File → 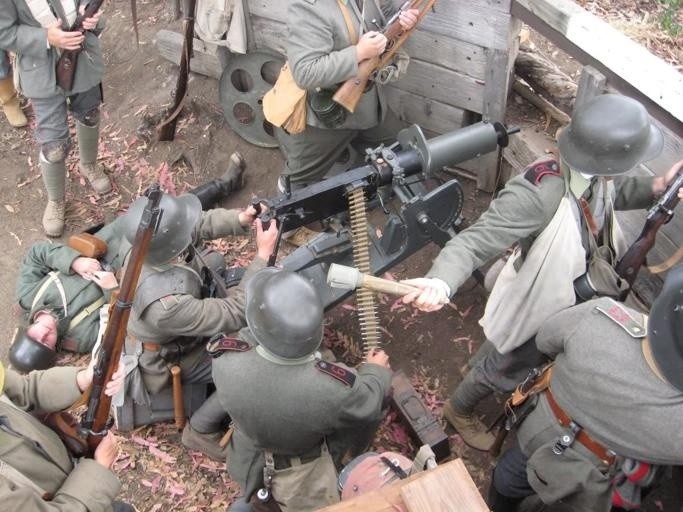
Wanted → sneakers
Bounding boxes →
[78,160,112,195]
[487,467,526,512]
[181,419,228,463]
[280,225,321,247]
[442,398,497,452]
[42,198,65,236]
[460,363,471,380]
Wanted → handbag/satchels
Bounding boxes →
[477,189,588,355]
[263,437,341,512]
[91,304,153,409]
[526,419,614,512]
[261,60,308,135]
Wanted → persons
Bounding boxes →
[402,95,683,511]
[0,192,395,511]
[1,0,112,236]
[273,1,421,246]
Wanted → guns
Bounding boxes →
[158,1,197,140]
[75,184,165,456]
[612,165,683,304]
[331,0,437,114]
[54,0,104,93]
[249,115,521,313]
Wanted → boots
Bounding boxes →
[187,150,246,211]
[0,74,28,127]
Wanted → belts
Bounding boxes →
[126,334,162,351]
[543,385,620,468]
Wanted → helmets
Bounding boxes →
[244,265,326,363]
[646,261,683,393]
[556,93,665,177]
[122,192,202,267]
[8,325,55,372]
